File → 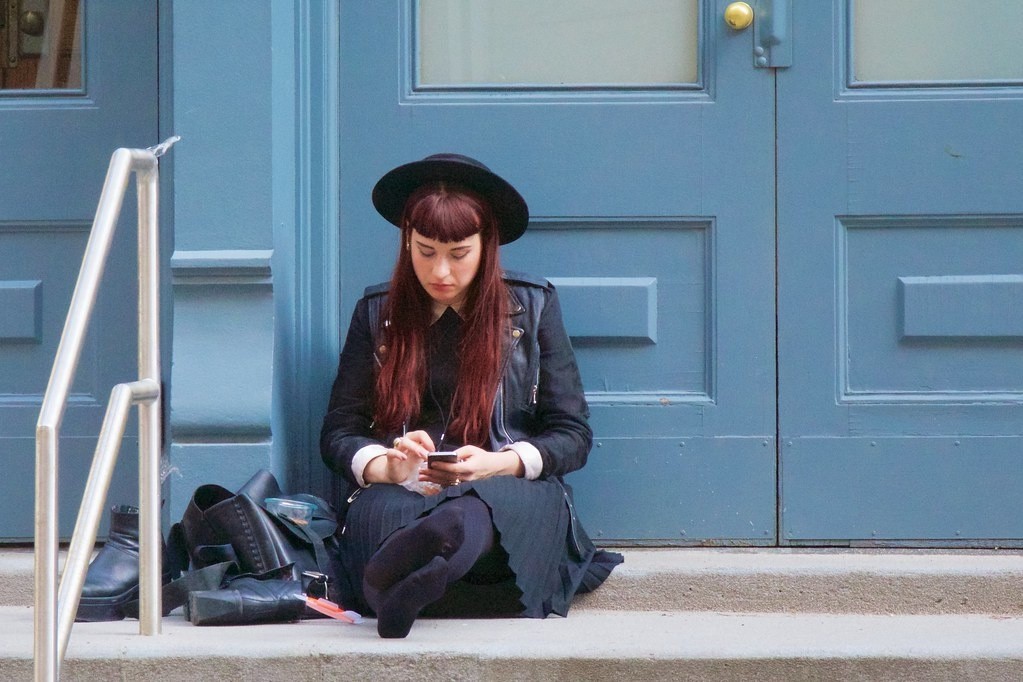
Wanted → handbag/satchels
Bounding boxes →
[168,472,356,613]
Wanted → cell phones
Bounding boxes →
[428,450,458,476]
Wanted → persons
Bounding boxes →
[320,154,624,639]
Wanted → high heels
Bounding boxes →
[182,562,307,625]
[74,505,172,622]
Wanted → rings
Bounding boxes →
[393,438,400,447]
[450,472,461,486]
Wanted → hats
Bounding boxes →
[371,153,529,246]
